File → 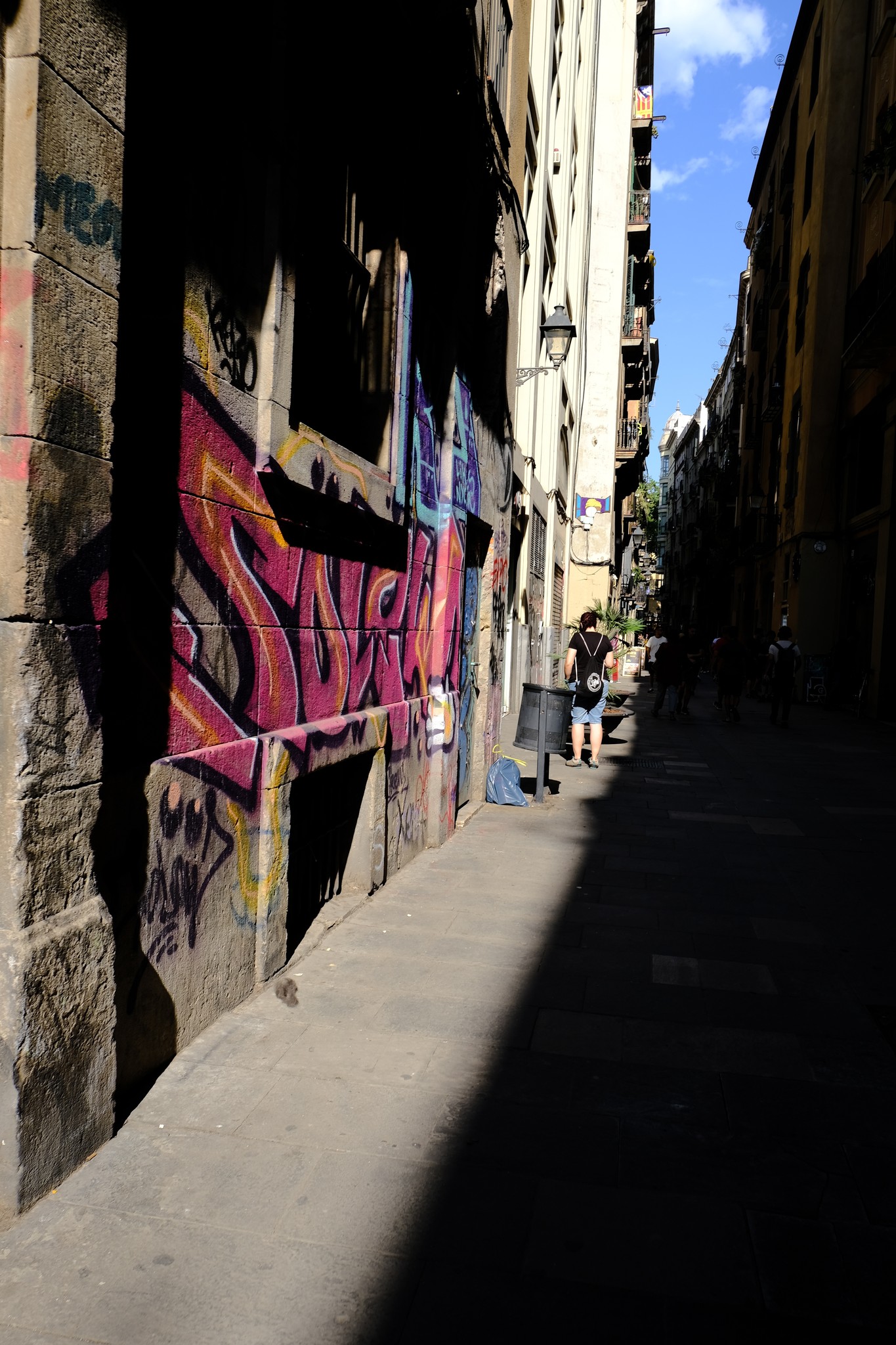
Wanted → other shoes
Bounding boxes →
[675,708,681,714]
[713,701,723,710]
[647,687,654,693]
[651,708,658,717]
[681,707,691,715]
[668,713,677,721]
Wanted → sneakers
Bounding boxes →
[566,756,582,768]
[588,757,599,769]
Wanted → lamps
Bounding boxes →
[636,551,651,566]
[513,303,577,385]
[625,524,645,553]
[641,570,653,581]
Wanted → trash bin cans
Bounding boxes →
[513,682,577,754]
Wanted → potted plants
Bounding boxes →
[544,596,647,743]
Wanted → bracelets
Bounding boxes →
[564,679,569,682]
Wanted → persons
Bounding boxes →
[638,624,802,727]
[564,611,613,769]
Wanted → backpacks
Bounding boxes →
[772,641,797,680]
[575,632,605,700]
